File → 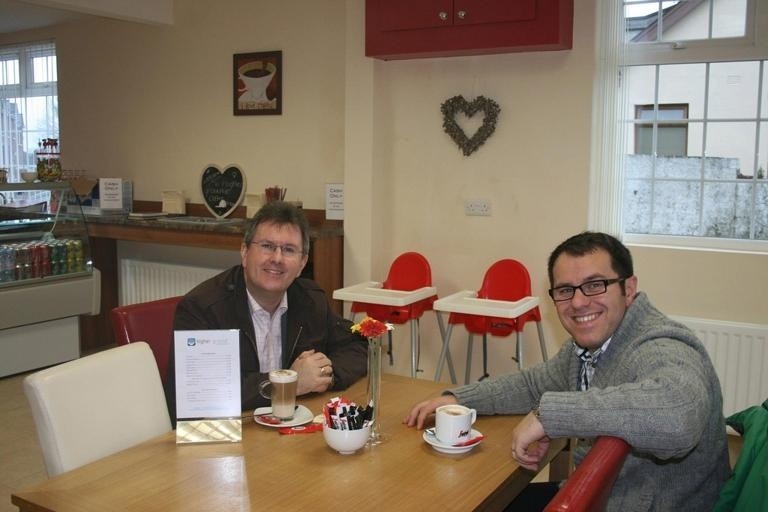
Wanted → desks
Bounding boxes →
[70,206,343,321]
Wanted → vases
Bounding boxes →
[367,336,383,439]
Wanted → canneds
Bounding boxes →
[0,239,93,282]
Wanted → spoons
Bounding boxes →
[232,404,298,421]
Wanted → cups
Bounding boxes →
[434,404,477,441]
[258,368,298,419]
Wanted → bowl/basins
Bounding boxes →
[321,419,375,454]
[21,172,38,183]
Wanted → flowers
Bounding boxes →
[348,316,389,430]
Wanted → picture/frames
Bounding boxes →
[231,50,283,116]
[161,188,186,216]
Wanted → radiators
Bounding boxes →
[659,312,768,435]
[117,257,226,309]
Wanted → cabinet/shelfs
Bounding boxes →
[363,0,575,64]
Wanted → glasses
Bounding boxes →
[547,276,625,303]
[244,238,305,258]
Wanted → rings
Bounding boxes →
[320,367,326,375]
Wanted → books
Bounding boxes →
[128,212,168,217]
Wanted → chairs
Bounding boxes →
[432,258,547,385]
[332,250,458,385]
[498,430,629,511]
[21,340,174,478]
[108,293,190,384]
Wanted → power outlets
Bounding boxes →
[465,200,490,216]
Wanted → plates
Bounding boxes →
[253,403,313,429]
[422,427,482,453]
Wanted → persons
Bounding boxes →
[401,232,735,512]
[164,202,371,428]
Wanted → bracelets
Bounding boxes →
[531,403,543,422]
